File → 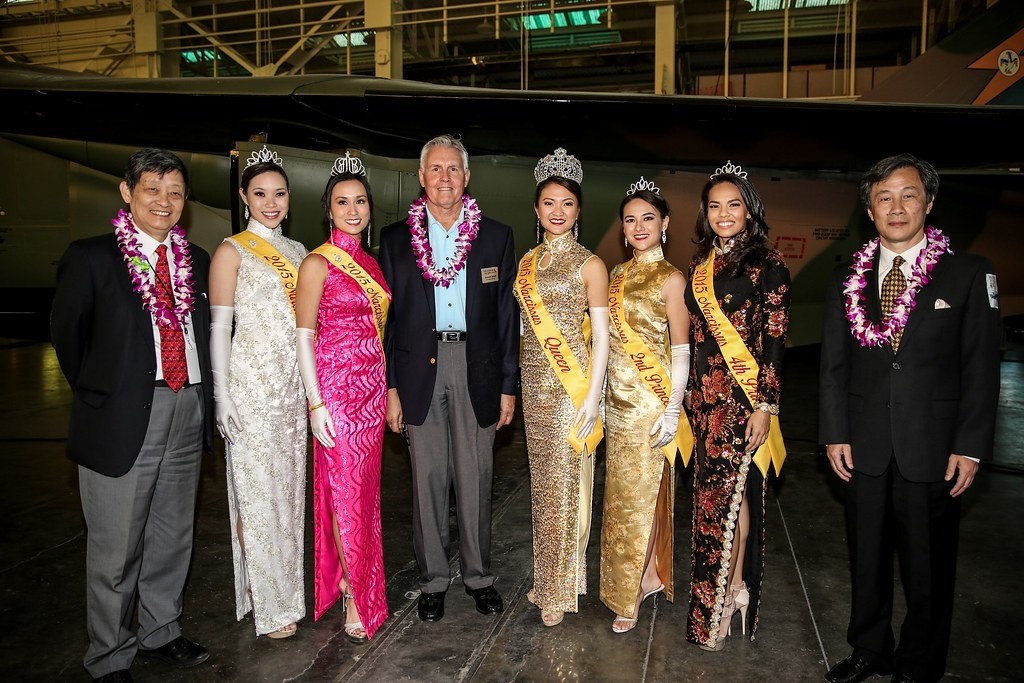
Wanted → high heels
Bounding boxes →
[247,590,255,619]
[339,578,351,612]
[730,581,749,635]
[612,591,644,633]
[699,587,736,651]
[344,594,366,642]
[643,584,665,608]
[266,622,297,638]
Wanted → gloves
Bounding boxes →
[574,307,609,439]
[295,328,336,448]
[209,306,242,444]
[650,344,690,449]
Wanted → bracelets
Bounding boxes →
[309,403,324,411]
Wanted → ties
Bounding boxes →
[881,255,909,356]
[156,244,188,392]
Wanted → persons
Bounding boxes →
[513,147,608,625]
[819,155,999,683]
[210,145,309,638]
[52,149,210,683]
[379,137,519,622]
[600,177,692,634]
[297,153,391,642]
[683,161,791,650]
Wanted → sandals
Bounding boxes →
[541,609,564,627]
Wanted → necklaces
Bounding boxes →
[112,210,195,327]
[843,225,950,345]
[409,194,481,287]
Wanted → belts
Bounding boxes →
[437,331,466,342]
[154,379,201,389]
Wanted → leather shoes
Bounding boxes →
[465,586,503,616]
[891,673,932,683]
[418,592,446,622]
[138,637,210,666]
[94,669,133,683]
[825,655,893,683]
